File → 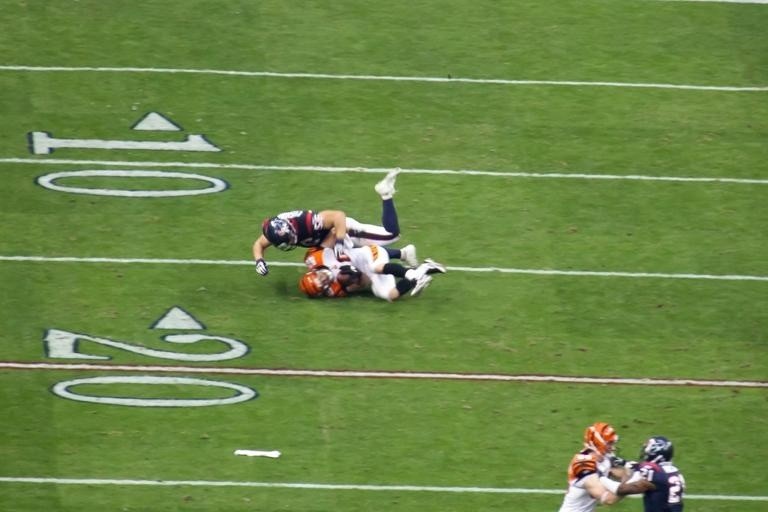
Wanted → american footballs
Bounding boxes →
[336,270,360,285]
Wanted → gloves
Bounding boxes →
[255,259,269,276]
[333,240,343,260]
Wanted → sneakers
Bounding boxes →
[402,244,417,269]
[410,274,431,297]
[421,259,445,273]
[374,167,401,196]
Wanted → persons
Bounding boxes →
[558,422,644,512]
[599,434,686,512]
[252,167,447,304]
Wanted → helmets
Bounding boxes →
[261,216,297,251]
[638,436,672,464]
[298,265,334,299]
[582,422,618,460]
